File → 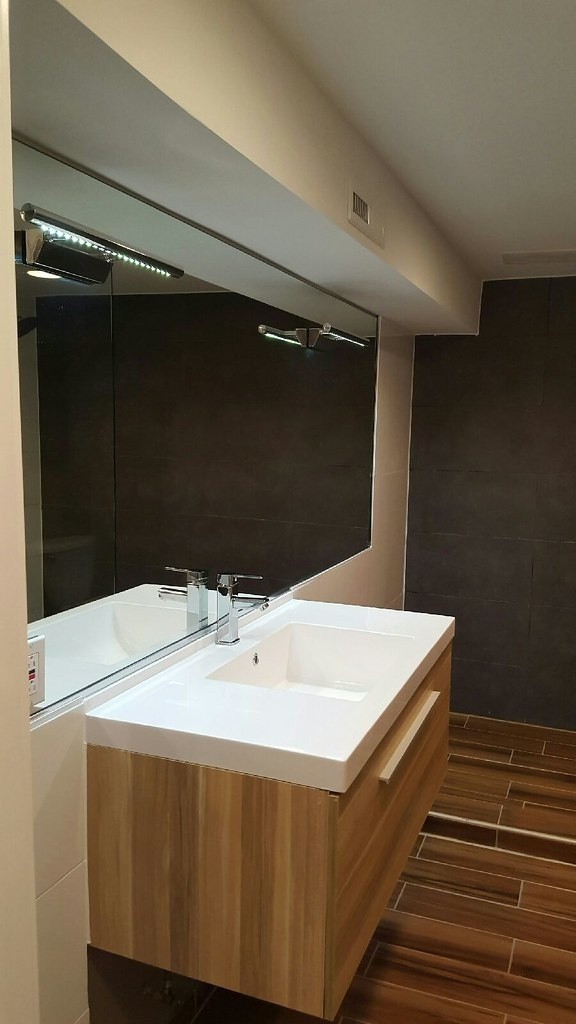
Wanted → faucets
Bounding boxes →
[215,574,269,646]
[158,567,208,636]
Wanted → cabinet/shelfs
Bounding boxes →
[88,642,452,1021]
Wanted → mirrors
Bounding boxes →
[12,137,380,717]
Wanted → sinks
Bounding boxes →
[204,621,417,703]
[27,601,215,667]
